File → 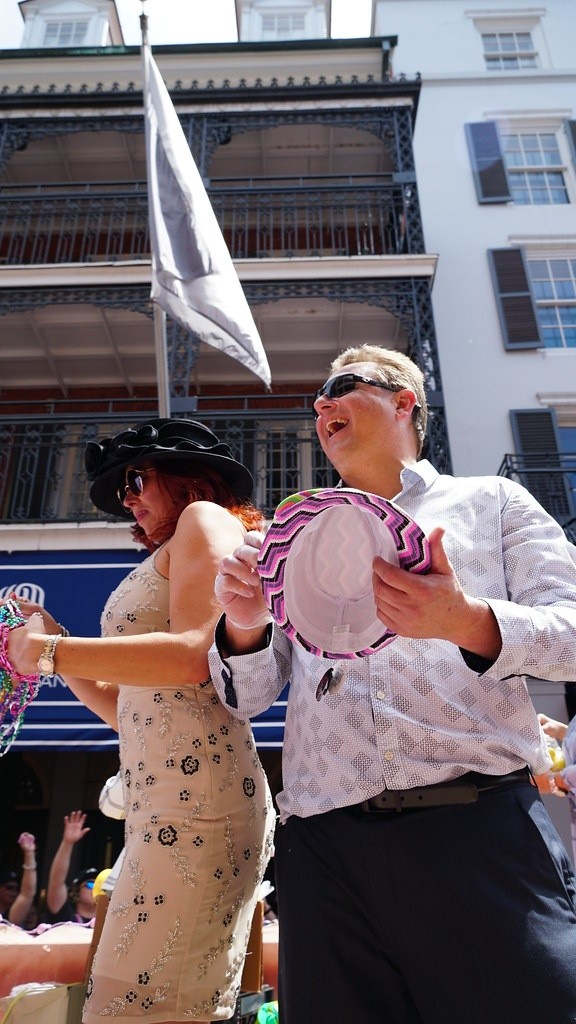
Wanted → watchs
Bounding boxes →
[37,634,61,678]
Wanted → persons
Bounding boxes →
[207,345,576,1023]
[8,415,276,1024]
[48,810,104,931]
[17,904,39,932]
[0,833,38,928]
[533,710,576,875]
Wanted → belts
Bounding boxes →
[343,767,532,813]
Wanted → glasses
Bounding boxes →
[2,884,20,891]
[116,466,158,514]
[310,373,423,420]
[78,880,94,888]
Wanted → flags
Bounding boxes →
[145,42,273,391]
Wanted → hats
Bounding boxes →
[0,870,20,886]
[71,865,100,887]
[253,488,432,662]
[83,416,254,521]
